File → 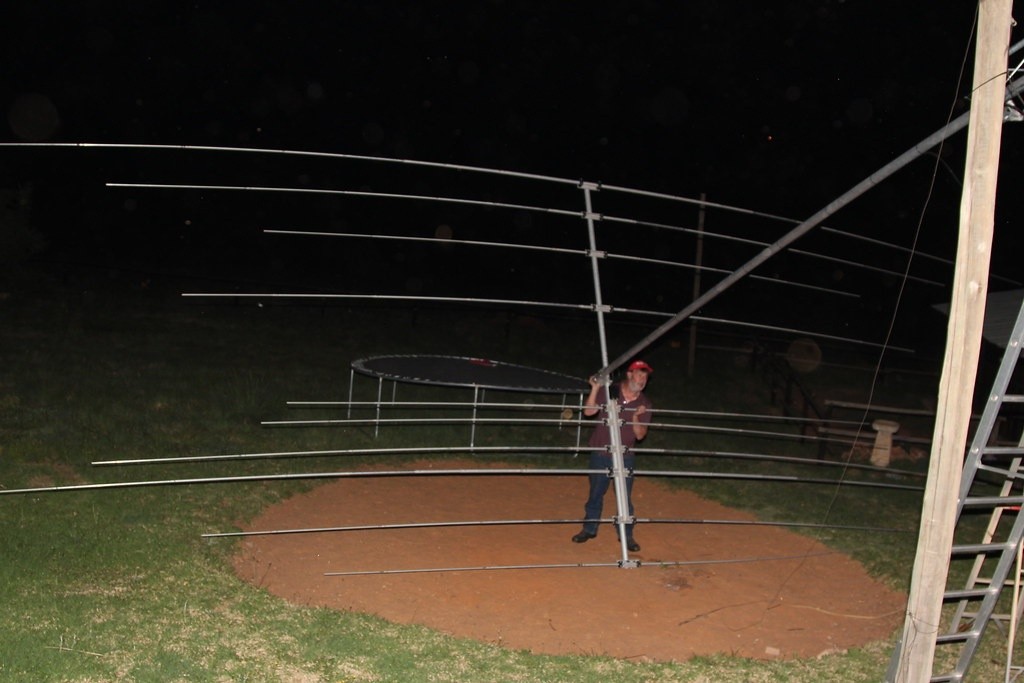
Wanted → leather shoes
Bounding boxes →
[618,538,640,551]
[572,531,596,543]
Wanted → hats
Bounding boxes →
[628,361,652,373]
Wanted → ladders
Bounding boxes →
[884,300,1024,683]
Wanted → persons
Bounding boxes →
[583,361,653,554]
